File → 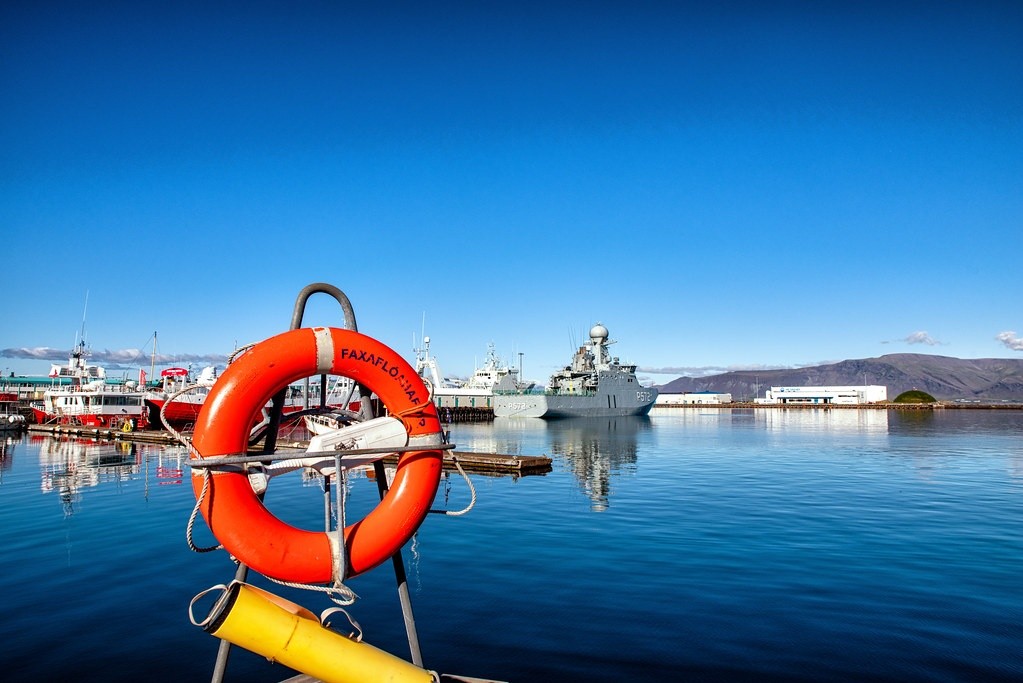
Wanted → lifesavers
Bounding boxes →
[189,326,444,585]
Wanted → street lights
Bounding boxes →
[517,352,526,383]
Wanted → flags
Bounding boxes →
[140,369,147,385]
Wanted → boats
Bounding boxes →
[0,285,535,438]
[491,320,659,419]
